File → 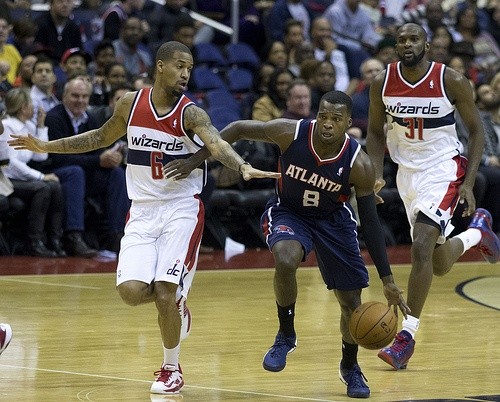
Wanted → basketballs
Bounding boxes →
[349,301,398,350]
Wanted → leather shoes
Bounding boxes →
[46,238,67,257]
[15,240,57,258]
[62,232,97,258]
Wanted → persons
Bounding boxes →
[5,41,282,395]
[365,23,499,368]
[0,0,496,255]
[161,90,410,398]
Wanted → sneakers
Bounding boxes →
[378,330,415,369]
[469,208,500,264]
[176,296,191,338]
[150,362,184,394]
[338,359,370,398]
[262,328,298,372]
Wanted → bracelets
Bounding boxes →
[236,161,252,174]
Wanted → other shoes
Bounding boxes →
[0,323,12,356]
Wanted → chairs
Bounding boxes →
[0,0,488,261]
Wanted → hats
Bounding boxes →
[62,47,93,65]
